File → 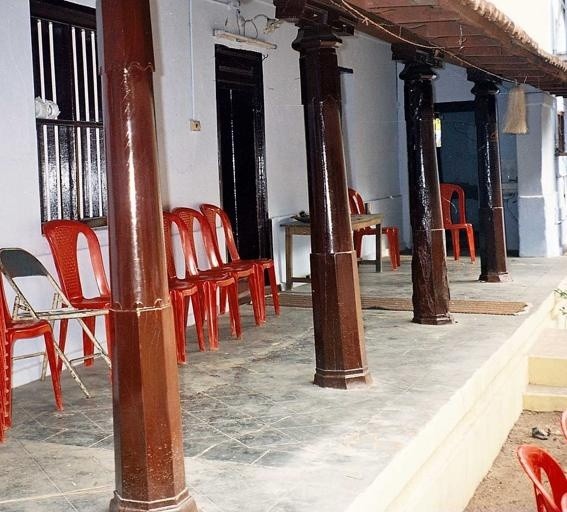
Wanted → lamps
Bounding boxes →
[244,14,285,35]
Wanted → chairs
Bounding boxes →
[0,247,112,399]
[42,219,113,375]
[0,275,64,442]
[348,187,400,271]
[517,445,567,512]
[560,411,567,440]
[440,183,475,262]
[162,204,279,364]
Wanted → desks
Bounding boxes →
[279,213,383,290]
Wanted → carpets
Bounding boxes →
[251,292,529,316]
